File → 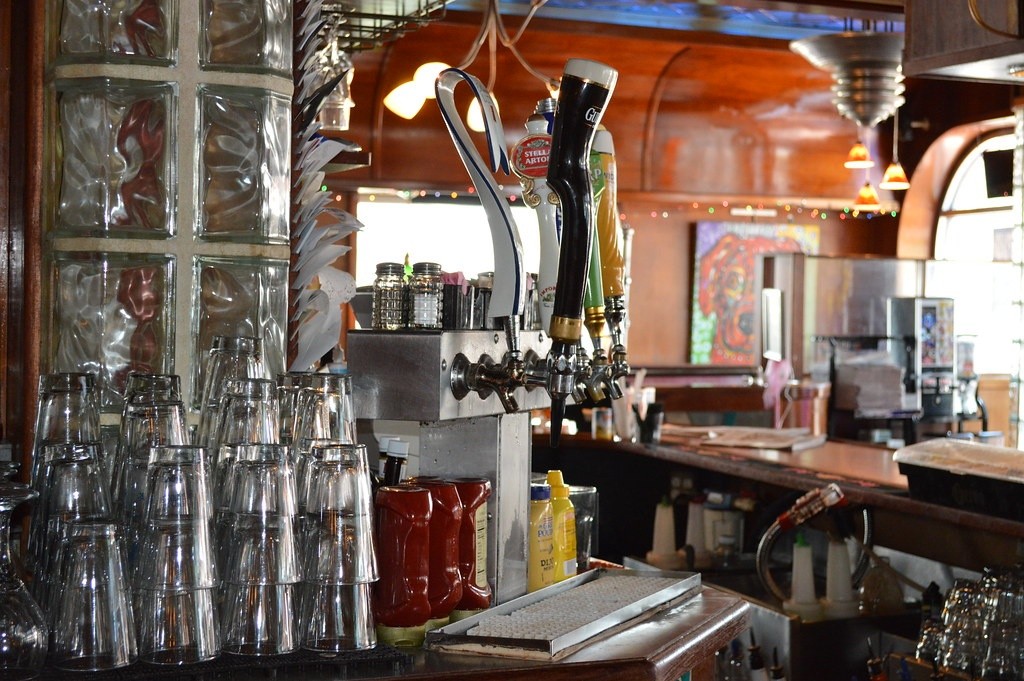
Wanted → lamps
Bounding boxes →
[382,0,560,133]
[788,15,912,211]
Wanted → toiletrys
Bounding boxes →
[527,469,578,592]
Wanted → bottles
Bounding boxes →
[826,531,852,602]
[686,492,705,552]
[408,262,444,329]
[728,631,787,681]
[370,435,414,500]
[791,531,815,604]
[654,496,676,555]
[372,263,406,329]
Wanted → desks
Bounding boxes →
[530,410,1024,565]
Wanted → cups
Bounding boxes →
[0,364,378,675]
[856,557,903,612]
[565,486,600,574]
[915,566,1024,681]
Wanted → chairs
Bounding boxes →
[974,378,1012,431]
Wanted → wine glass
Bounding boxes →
[320,4,355,109]
[319,26,353,86]
[321,14,350,131]
